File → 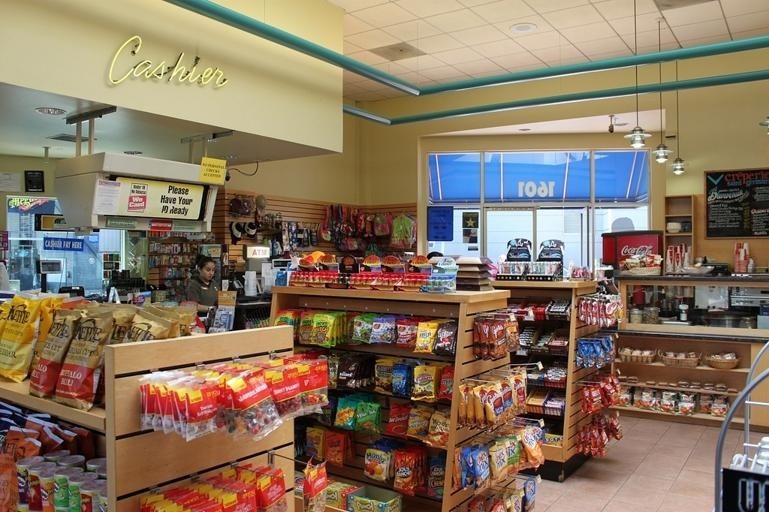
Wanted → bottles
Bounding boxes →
[182,243,191,253]
[496,261,561,276]
[290,269,455,289]
[12,448,108,512]
[166,266,173,278]
[626,284,694,324]
[738,316,756,329]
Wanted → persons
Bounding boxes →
[426,251,443,260]
[184,252,222,316]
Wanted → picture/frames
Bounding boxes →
[24,169,45,193]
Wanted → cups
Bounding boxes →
[732,242,755,274]
[109,269,145,291]
[680,221,692,230]
[665,243,691,273]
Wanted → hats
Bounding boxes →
[229,221,258,241]
[254,194,267,217]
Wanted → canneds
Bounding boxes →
[679,304,689,321]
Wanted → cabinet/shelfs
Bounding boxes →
[714,342,769,511]
[0,251,627,512]
[661,192,697,274]
[596,232,769,433]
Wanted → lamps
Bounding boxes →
[670,50,686,176]
[653,19,673,165]
[621,0,653,149]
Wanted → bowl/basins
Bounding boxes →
[754,266,768,273]
[666,222,681,233]
